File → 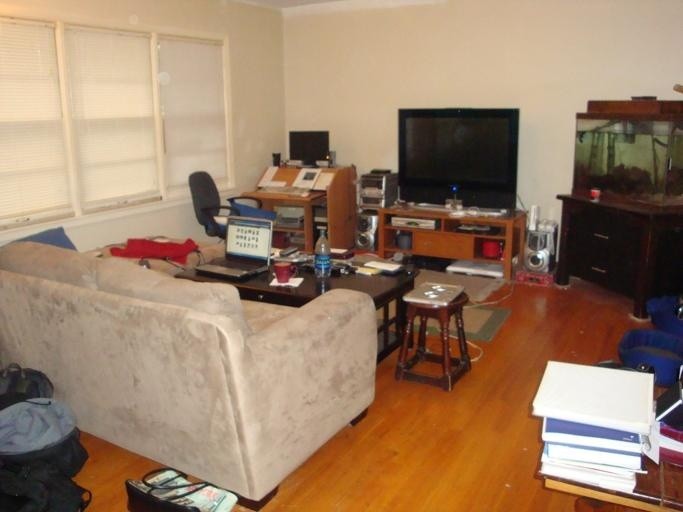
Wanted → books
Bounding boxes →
[533,359,682,492]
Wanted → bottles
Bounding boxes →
[312,229,331,278]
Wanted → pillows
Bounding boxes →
[7,226,78,252]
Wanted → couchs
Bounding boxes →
[0,240,379,510]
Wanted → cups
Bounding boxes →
[589,188,602,204]
[273,263,291,282]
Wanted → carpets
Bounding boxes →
[412,268,513,348]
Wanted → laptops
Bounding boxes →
[194,215,275,281]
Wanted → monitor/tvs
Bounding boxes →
[289,130,329,168]
[397,107,520,209]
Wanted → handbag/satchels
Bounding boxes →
[125,467,239,512]
[0,364,92,512]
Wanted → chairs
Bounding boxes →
[186,169,278,238]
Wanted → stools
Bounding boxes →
[391,282,474,395]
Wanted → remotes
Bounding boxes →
[279,246,299,256]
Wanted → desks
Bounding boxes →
[533,424,683,511]
[552,192,683,323]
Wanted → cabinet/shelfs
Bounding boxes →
[248,162,525,277]
